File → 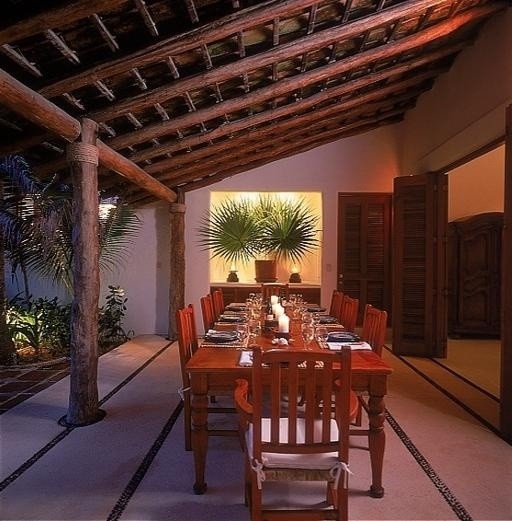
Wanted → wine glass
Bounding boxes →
[249,321,260,347]
[236,324,248,350]
[316,326,328,349]
[289,293,321,324]
[239,293,262,323]
[301,323,315,351]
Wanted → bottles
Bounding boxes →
[278,295,287,307]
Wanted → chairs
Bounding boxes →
[298,290,387,427]
[175,289,248,452]
[233,345,352,521]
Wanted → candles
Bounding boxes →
[270,295,289,332]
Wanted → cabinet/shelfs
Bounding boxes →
[210,284,321,311]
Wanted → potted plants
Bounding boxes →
[193,192,323,282]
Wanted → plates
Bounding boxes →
[225,305,246,311]
[308,307,326,313]
[328,331,360,342]
[203,330,239,342]
[220,314,244,323]
[315,314,338,324]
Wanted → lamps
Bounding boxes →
[288,261,302,283]
[226,261,240,282]
